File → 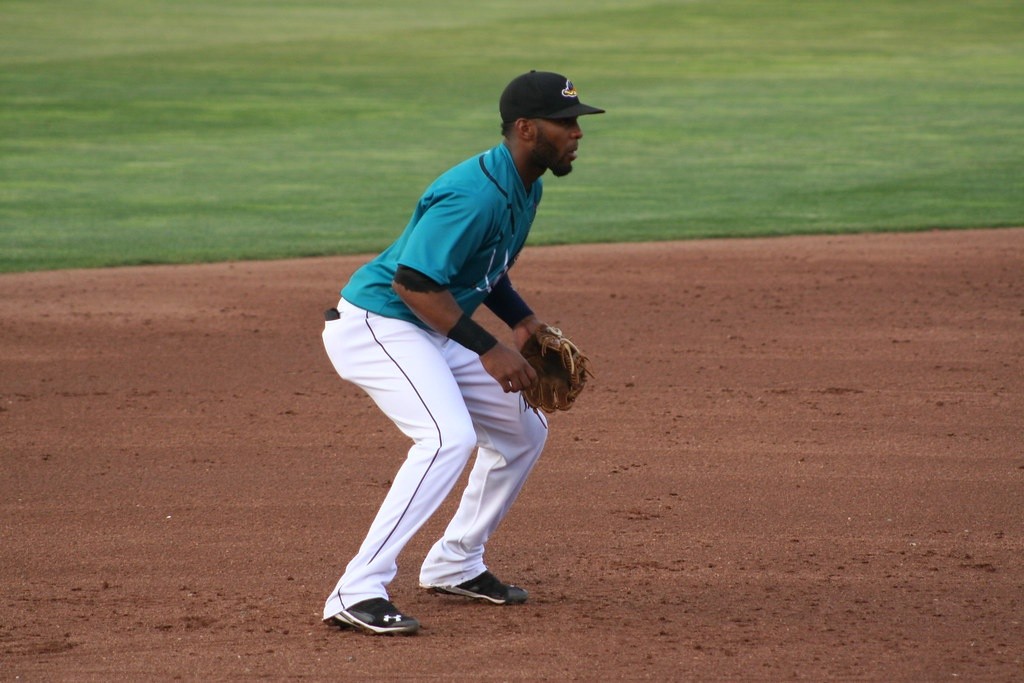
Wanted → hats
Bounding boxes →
[500,70,605,123]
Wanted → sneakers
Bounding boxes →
[434,569,528,604]
[322,598,419,636]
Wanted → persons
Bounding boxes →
[322,69,606,636]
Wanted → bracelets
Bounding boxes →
[447,312,499,356]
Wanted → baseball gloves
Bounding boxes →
[516,322,588,415]
